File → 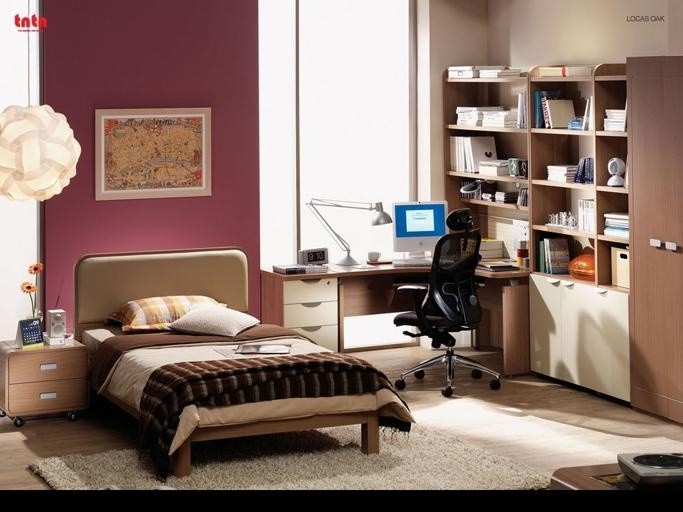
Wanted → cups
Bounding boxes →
[368,251,381,262]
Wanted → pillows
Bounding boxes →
[106,295,260,337]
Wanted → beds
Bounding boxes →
[75,246,416,477]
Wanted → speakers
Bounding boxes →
[48,308,66,338]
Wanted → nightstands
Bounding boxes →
[0,338,90,428]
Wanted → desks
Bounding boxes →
[551,463,683,490]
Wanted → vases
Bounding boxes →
[26,309,44,323]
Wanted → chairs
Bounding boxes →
[394,208,501,397]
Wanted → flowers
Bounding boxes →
[21,262,43,318]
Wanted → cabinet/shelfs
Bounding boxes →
[530,64,630,404]
[260,261,532,378]
[442,69,530,268]
[626,57,683,425]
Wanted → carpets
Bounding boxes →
[28,423,551,490]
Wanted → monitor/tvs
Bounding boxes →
[392,200,449,258]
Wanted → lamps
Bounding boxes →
[1,1,82,201]
[305,198,392,265]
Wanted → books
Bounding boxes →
[272,264,327,275]
[447,66,630,291]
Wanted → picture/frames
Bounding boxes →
[95,108,211,201]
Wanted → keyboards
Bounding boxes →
[392,258,432,267]
